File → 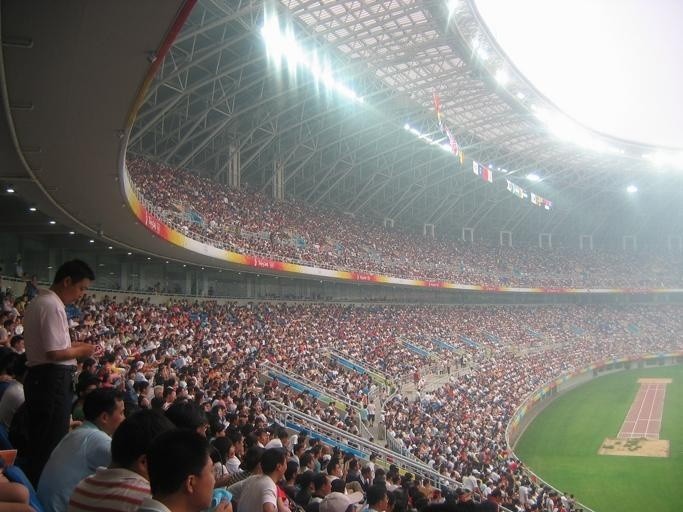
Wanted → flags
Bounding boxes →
[432,92,464,164]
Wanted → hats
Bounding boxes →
[317,491,366,512]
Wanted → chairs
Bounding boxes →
[5,464,45,512]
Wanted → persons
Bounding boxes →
[127,155,681,261]
[0,260,683,512]
